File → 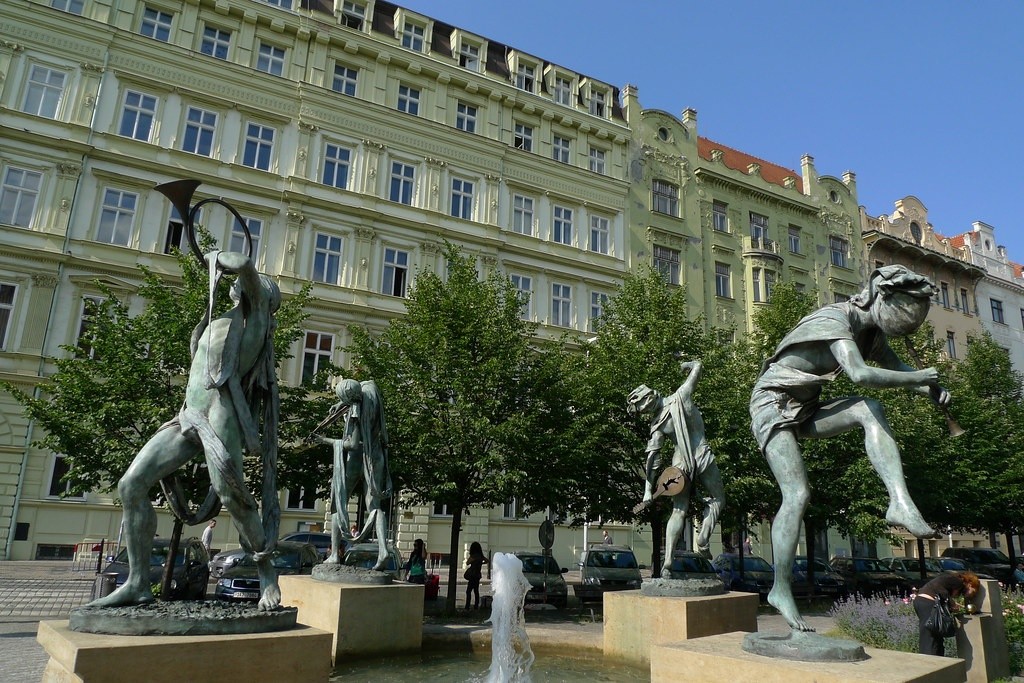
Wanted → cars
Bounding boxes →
[770,555,846,601]
[213,541,324,606]
[514,551,569,611]
[878,546,1024,592]
[208,532,371,577]
[709,552,774,596]
[651,550,722,583]
[828,556,909,601]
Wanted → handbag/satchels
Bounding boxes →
[925,594,957,637]
[463,568,482,580]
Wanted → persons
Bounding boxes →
[85,248,285,611]
[462,541,489,613]
[200,518,216,558]
[403,538,432,590]
[749,263,950,632]
[602,529,614,556]
[627,361,727,578]
[323,540,358,565]
[312,379,393,571]
[914,572,980,656]
[732,538,752,554]
[1014,562,1024,596]
[351,525,361,539]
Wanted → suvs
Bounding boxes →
[341,543,404,581]
[90,534,210,604]
[577,544,646,602]
